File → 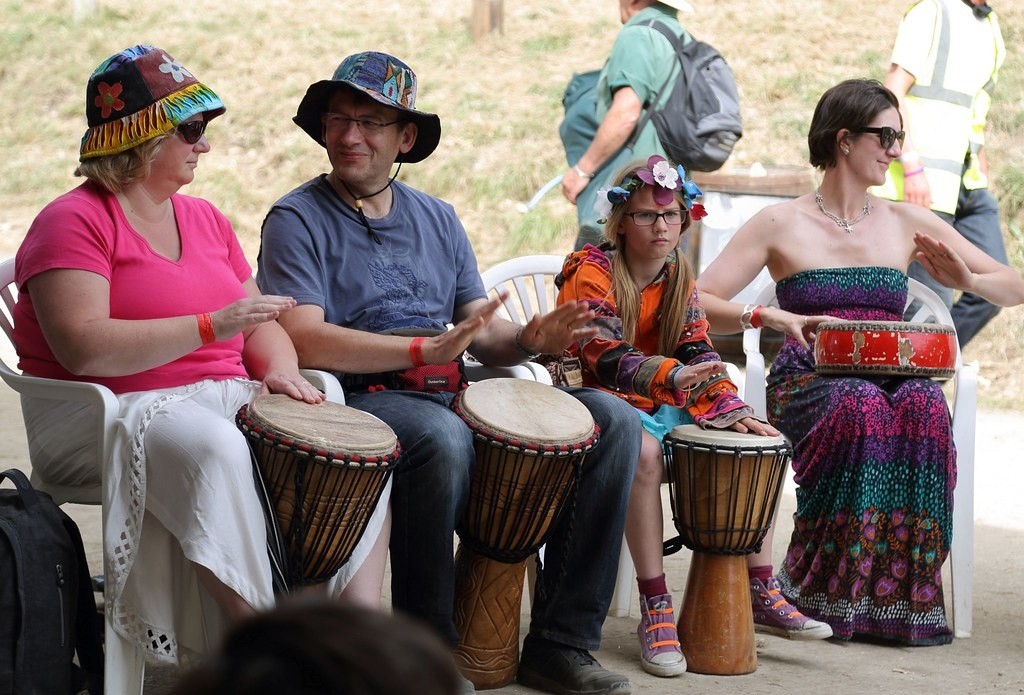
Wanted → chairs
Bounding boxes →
[0,257,346,695]
[468,256,741,618]
[743,276,979,640]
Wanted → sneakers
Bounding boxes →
[515,632,634,695]
[635,593,687,678]
[749,576,834,641]
[443,649,477,694]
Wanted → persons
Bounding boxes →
[870,0,1008,388]
[254,50,642,695]
[694,78,1024,647]
[562,0,695,252]
[12,42,392,668]
[554,154,833,676]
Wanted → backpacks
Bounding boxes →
[0,466,105,695]
[632,18,745,173]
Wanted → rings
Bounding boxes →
[943,254,947,258]
[568,325,572,331]
[805,317,807,325]
[303,382,309,384]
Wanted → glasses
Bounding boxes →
[623,209,689,227]
[851,126,905,151]
[320,111,402,134]
[168,120,212,145]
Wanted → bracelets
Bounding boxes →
[740,304,763,329]
[409,337,427,367]
[669,365,701,391]
[515,325,541,359]
[904,167,923,177]
[196,313,216,346]
[575,166,590,178]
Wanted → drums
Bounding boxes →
[663,423,787,675]
[237,392,401,608]
[452,378,602,686]
[814,319,956,379]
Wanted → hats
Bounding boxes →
[657,0,695,13]
[78,43,228,162]
[291,50,443,164]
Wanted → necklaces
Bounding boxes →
[815,190,871,234]
